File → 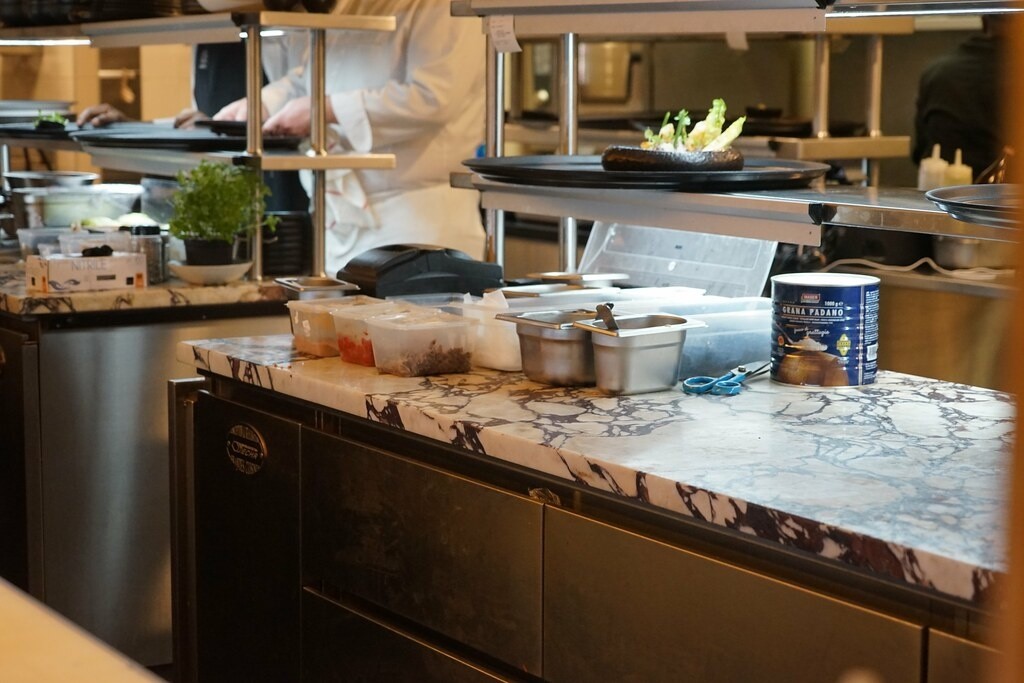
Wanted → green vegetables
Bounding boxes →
[645,100,746,152]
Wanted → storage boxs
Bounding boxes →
[274,276,775,398]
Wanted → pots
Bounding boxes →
[12,184,146,230]
[3,171,101,191]
[935,234,1018,269]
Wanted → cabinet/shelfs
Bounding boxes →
[0,317,1021,683]
[1,0,1024,289]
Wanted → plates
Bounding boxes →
[167,259,254,284]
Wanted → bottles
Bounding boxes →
[938,149,972,187]
[974,144,1015,185]
[917,144,948,191]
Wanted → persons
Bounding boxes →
[77,0,313,272]
[910,14,1006,190]
[211,0,500,281]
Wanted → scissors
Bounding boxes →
[682,361,770,396]
